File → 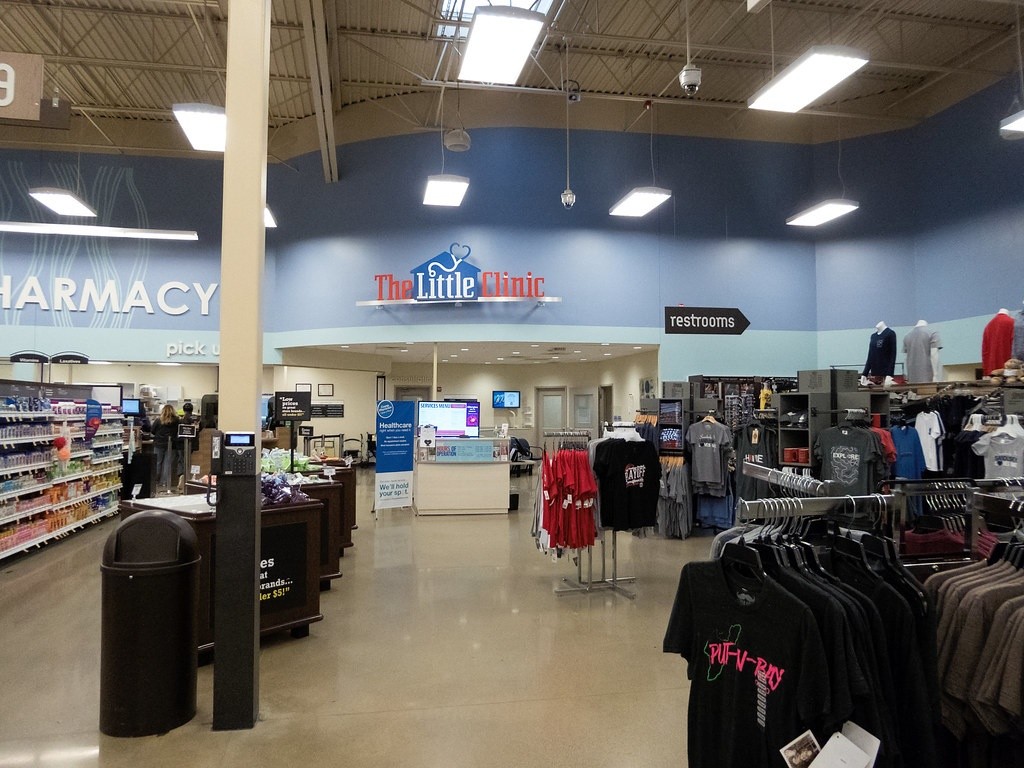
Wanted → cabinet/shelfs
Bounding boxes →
[0,410,125,561]
[690,381,724,423]
[777,369,834,467]
[833,369,890,434]
[657,381,691,450]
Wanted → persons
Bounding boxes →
[901,319,943,382]
[861,321,897,387]
[982,301,1024,381]
[151,403,198,490]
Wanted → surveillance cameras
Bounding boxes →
[561,190,576,210]
[678,63,701,95]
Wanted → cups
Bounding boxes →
[502,423,508,437]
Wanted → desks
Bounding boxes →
[118,460,363,668]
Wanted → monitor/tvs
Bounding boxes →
[492,391,520,408]
[418,401,481,439]
[122,399,140,414]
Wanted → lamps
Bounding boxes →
[443,79,472,152]
[173,0,226,152]
[264,204,278,228]
[0,221,199,240]
[423,95,469,207]
[998,94,1024,141]
[746,4,870,113]
[28,110,98,216]
[455,6,545,86]
[784,119,859,227]
[609,95,672,216]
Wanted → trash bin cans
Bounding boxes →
[99,509,202,738]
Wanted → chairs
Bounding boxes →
[342,432,376,468]
[511,437,543,478]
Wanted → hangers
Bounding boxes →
[543,431,585,458]
[603,421,645,442]
[702,410,717,423]
[961,396,1024,438]
[633,409,657,427]
[838,409,873,431]
[923,385,955,414]
[659,451,686,467]
[721,467,1024,583]
[897,418,909,429]
[730,408,777,436]
